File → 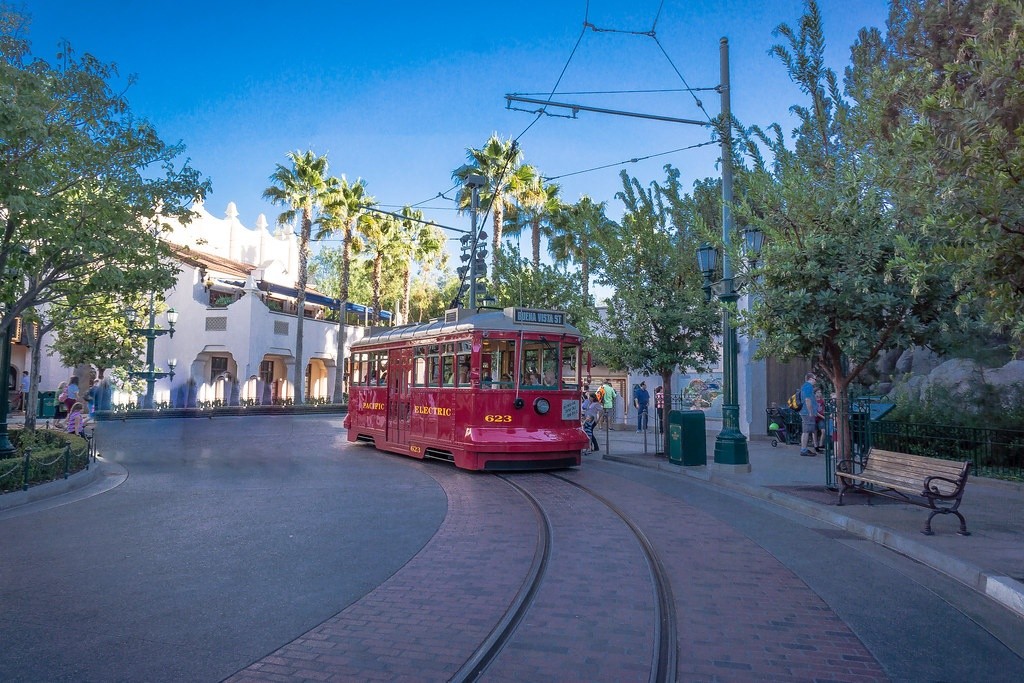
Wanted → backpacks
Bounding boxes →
[58,384,70,402]
[787,389,803,411]
[594,386,606,403]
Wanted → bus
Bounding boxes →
[339,141,592,473]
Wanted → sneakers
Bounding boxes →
[800,449,817,456]
[813,445,826,454]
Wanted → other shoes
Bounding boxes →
[599,427,604,431]
[642,429,652,434]
[584,450,592,455]
[636,429,641,434]
[608,427,615,432]
[594,449,600,453]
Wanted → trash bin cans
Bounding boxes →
[667,410,707,466]
[36,391,58,419]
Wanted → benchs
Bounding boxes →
[834,445,973,536]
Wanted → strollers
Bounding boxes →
[765,407,798,447]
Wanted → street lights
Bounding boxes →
[126,217,178,408]
[457,174,488,308]
[678,36,765,464]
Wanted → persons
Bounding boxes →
[52,381,68,429]
[635,381,651,434]
[500,373,513,389]
[371,369,387,383]
[448,361,470,383]
[582,392,604,456]
[17,371,30,410]
[88,379,100,416]
[64,375,82,413]
[656,386,664,434]
[597,380,616,431]
[798,373,825,457]
[67,402,90,439]
[545,369,557,386]
[525,372,541,385]
